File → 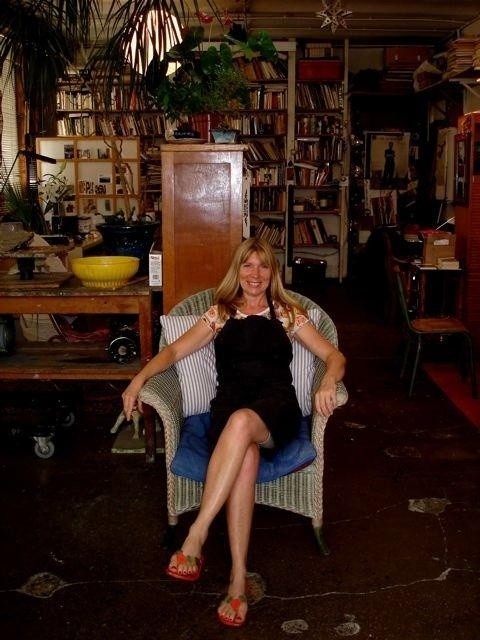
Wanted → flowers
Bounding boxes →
[34,158,77,216]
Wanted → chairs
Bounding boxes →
[136,281,351,561]
[392,270,475,399]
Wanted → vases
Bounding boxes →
[50,213,81,241]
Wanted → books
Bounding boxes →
[50,90,197,215]
[434,257,460,270]
[217,43,344,247]
[442,36,480,81]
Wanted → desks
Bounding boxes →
[391,252,466,348]
[0,272,160,465]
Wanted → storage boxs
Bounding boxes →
[147,241,162,289]
[421,233,458,268]
[298,58,343,83]
[384,43,433,72]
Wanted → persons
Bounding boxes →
[114,236,348,630]
[381,141,396,181]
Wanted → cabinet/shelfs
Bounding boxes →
[23,27,351,287]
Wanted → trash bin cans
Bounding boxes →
[292,256,327,303]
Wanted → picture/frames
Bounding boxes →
[453,133,470,204]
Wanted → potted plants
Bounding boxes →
[0,2,235,278]
[199,65,253,145]
[139,21,283,143]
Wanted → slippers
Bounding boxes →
[166,551,205,581]
[216,594,248,627]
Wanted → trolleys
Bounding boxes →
[0,380,77,460]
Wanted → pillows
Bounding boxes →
[159,307,325,421]
